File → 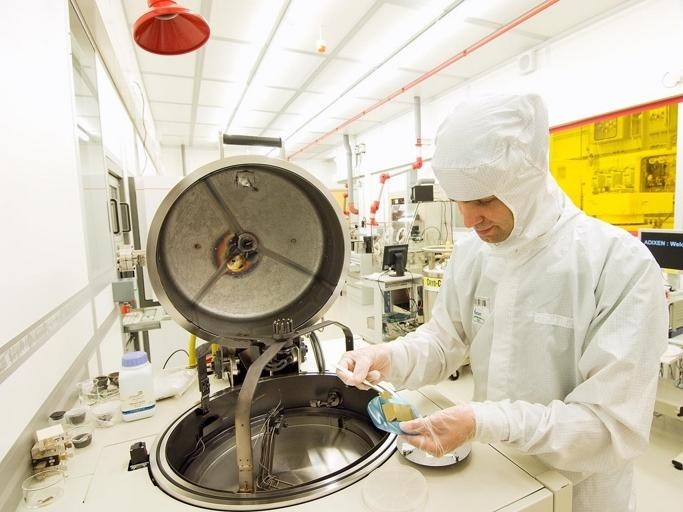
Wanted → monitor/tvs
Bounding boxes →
[637,229,682,274]
[382,244,408,277]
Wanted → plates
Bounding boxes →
[397,437,472,468]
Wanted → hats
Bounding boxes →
[426,92,583,271]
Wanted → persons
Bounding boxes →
[336,92,670,510]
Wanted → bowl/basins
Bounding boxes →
[20,467,68,512]
[67,371,119,450]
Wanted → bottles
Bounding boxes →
[118,350,157,424]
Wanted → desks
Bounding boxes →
[357,270,423,344]
